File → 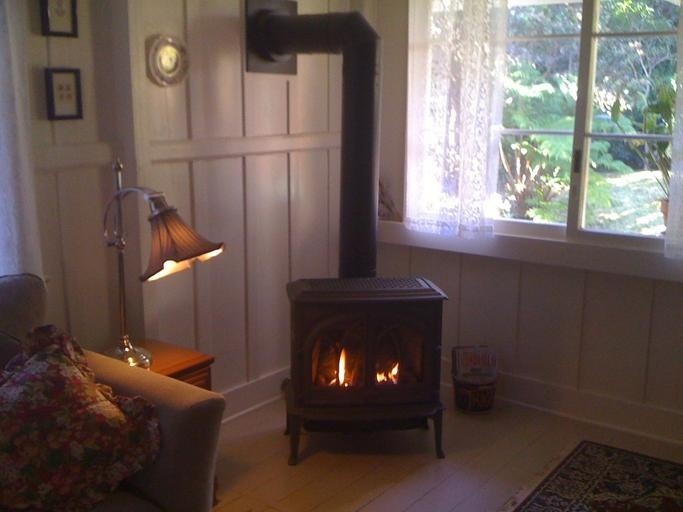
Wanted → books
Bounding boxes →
[453,345,496,374]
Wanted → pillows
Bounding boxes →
[1,321,162,510]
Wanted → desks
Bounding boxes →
[78,337,218,394]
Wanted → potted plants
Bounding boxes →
[609,74,671,234]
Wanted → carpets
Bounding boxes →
[510,436,682,512]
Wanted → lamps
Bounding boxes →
[96,157,226,376]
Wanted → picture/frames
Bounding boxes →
[40,0,78,37]
[45,68,83,120]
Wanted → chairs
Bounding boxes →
[0,269,229,512]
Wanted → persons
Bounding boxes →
[645,179,668,237]
